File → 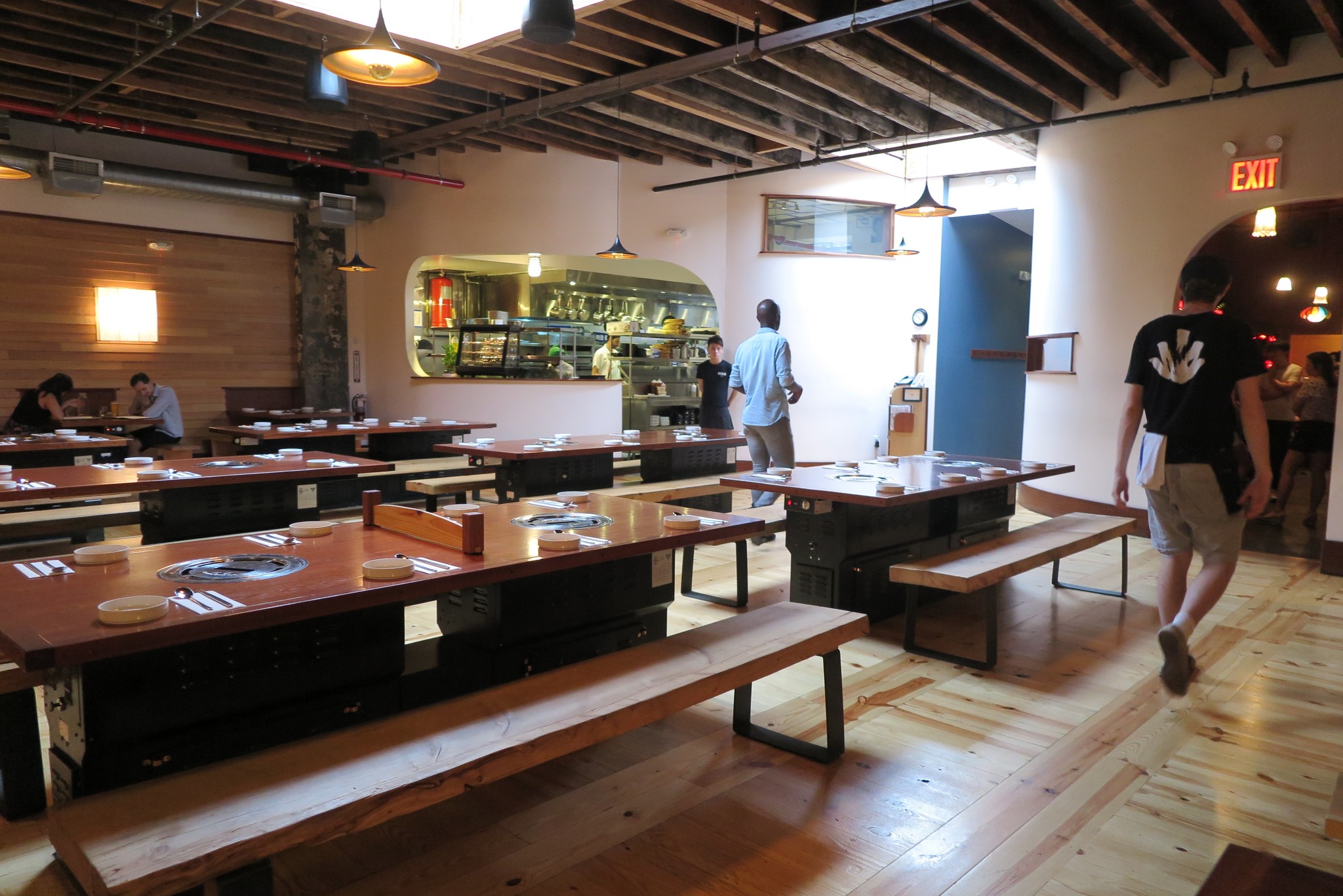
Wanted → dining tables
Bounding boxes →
[1,491,763,807]
[0,451,395,536]
[235,407,354,421]
[0,432,133,460]
[720,449,1076,622]
[435,427,748,498]
[208,416,496,460]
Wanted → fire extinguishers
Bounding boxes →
[431,272,452,327]
[352,392,368,422]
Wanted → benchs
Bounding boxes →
[46,602,868,895]
[889,512,1138,671]
[358,454,787,608]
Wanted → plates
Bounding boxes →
[650,415,670,426]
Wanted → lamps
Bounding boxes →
[587,64,638,260]
[338,217,377,273]
[1223,133,1284,193]
[1252,207,1276,237]
[884,132,920,256]
[322,1,440,87]
[894,1,957,217]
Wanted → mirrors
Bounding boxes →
[758,193,895,260]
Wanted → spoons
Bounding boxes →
[553,530,595,544]
[43,560,67,573]
[266,534,296,543]
[394,554,437,572]
[673,512,716,524]
[174,587,212,610]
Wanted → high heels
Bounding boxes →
[1303,515,1317,526]
[1257,513,1284,525]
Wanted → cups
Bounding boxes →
[672,348,680,359]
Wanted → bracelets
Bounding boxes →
[727,402,730,407]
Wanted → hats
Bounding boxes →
[607,334,620,339]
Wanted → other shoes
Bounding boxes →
[1160,655,1196,675]
[1159,624,1189,696]
[751,533,776,545]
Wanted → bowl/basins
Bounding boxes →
[289,521,333,537]
[445,318,526,329]
[0,405,459,492]
[538,533,581,551]
[73,544,129,564]
[657,386,667,396]
[476,426,746,452]
[362,558,414,580]
[97,595,169,624]
[443,504,480,518]
[662,514,701,529]
[767,450,1047,493]
[557,491,589,503]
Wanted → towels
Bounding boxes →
[1137,433,1168,493]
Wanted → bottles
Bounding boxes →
[691,383,702,398]
[651,379,666,394]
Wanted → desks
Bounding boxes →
[59,414,164,435]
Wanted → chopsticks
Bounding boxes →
[197,589,233,608]
[23,561,47,577]
[701,518,724,523]
[408,556,450,569]
[251,534,284,546]
[581,536,609,543]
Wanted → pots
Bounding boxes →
[549,293,650,330]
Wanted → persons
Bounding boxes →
[1259,340,1303,503]
[617,343,647,357]
[1257,352,1339,528]
[1111,254,1272,697]
[662,315,675,325]
[417,339,434,371]
[547,344,576,378]
[690,339,706,357]
[729,299,803,545]
[4,373,86,469]
[126,372,184,458]
[696,335,738,430]
[592,335,628,384]
